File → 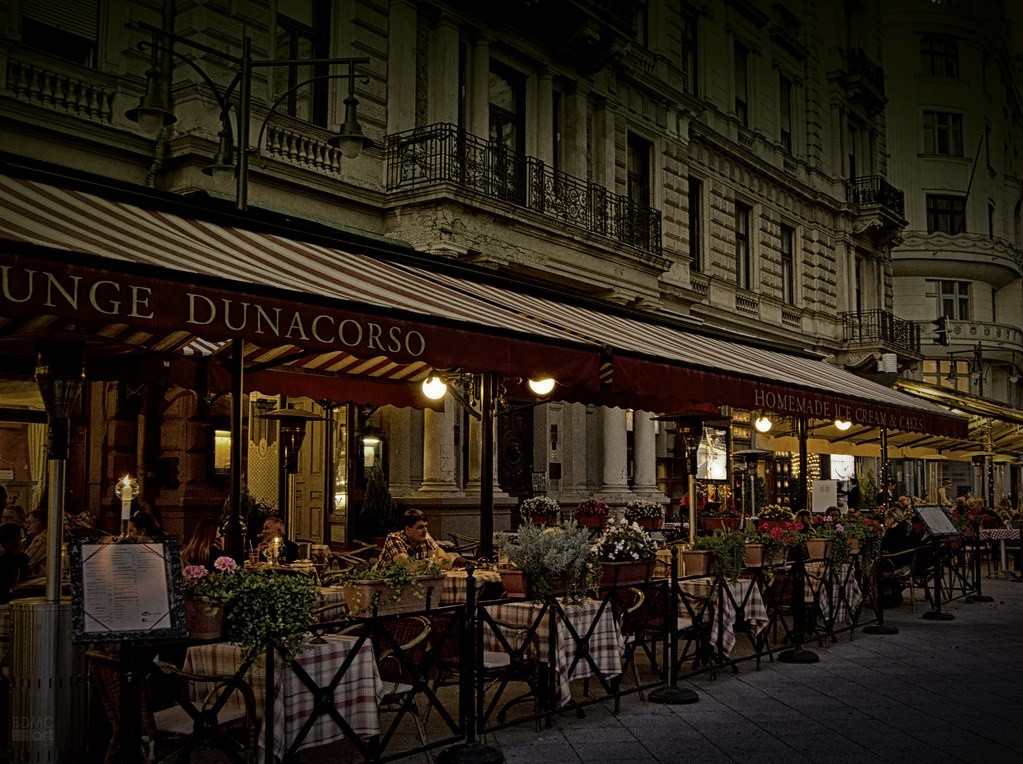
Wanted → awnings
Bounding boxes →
[0,172,970,443]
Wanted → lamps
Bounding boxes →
[259,403,333,474]
[214,429,232,475]
[114,473,140,538]
[362,438,381,467]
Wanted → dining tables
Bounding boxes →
[244,561,315,579]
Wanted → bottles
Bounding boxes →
[278,537,287,565]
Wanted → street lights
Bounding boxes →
[126,19,371,633]
[423,366,554,561]
[945,340,1019,510]
[755,409,851,519]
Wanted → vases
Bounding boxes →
[576,514,611,528]
[532,514,557,526]
[742,544,766,567]
[183,599,223,639]
[804,537,835,559]
[701,517,742,530]
[683,550,719,576]
[628,515,664,529]
[846,536,861,554]
[523,557,657,598]
[343,573,447,617]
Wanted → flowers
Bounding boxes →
[339,546,451,616]
[494,515,658,599]
[688,504,884,574]
[623,499,665,521]
[180,555,238,618]
[572,497,610,520]
[943,503,989,549]
[520,495,561,525]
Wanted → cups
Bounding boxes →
[249,549,259,568]
[428,550,438,562]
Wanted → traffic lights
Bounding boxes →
[931,315,947,345]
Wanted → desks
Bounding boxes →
[306,585,345,625]
[977,528,1023,579]
[804,562,864,649]
[180,632,384,764]
[439,569,502,603]
[482,597,627,728]
[669,576,770,671]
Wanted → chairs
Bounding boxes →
[351,539,383,572]
[414,612,542,739]
[336,616,436,764]
[447,532,499,562]
[731,564,802,662]
[877,545,938,614]
[625,582,717,681]
[583,588,648,702]
[86,649,261,763]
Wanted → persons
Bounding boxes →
[125,510,169,543]
[680,483,707,519]
[368,509,449,580]
[257,519,299,564]
[790,480,1007,597]
[182,519,228,571]
[0,484,49,603]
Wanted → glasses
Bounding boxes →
[1,515,14,521]
[134,511,141,520]
